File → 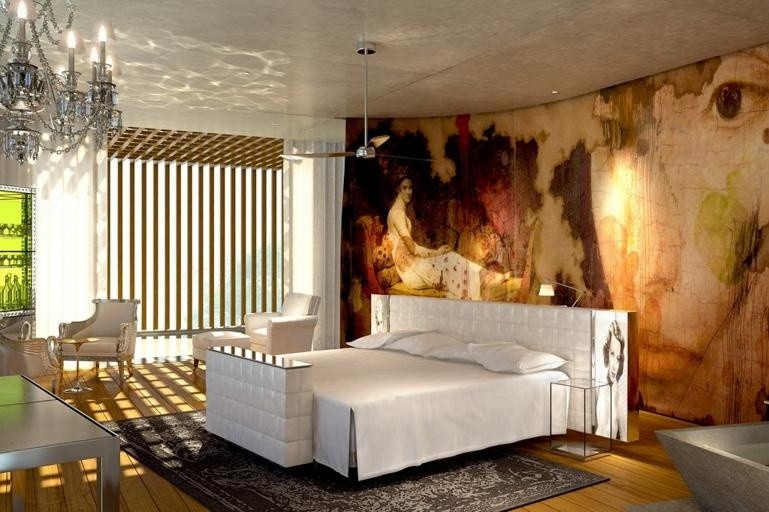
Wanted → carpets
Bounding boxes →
[98,408,612,511]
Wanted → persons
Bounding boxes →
[594,318,626,439]
[384,165,530,300]
[583,46,769,424]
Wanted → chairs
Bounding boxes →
[243,292,322,353]
[0,298,142,396]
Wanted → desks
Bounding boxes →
[0,372,126,511]
[547,376,616,463]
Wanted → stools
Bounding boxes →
[191,330,251,368]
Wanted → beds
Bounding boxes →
[277,292,592,489]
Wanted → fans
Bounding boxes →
[277,40,441,163]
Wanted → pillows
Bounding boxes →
[346,328,568,374]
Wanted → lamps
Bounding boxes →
[537,277,587,307]
[0,0,125,168]
